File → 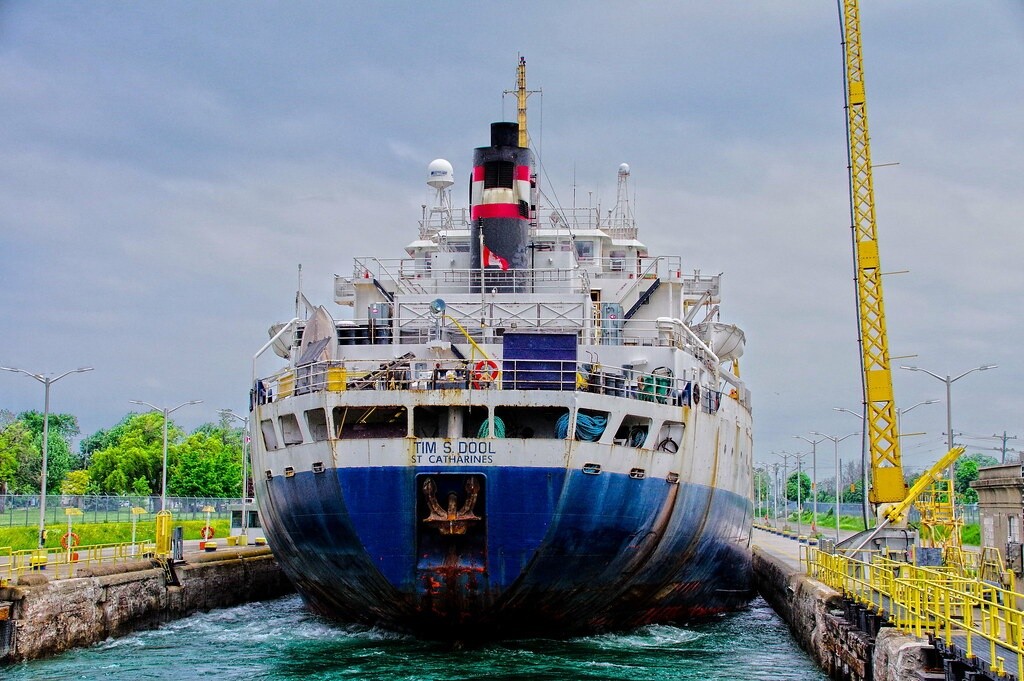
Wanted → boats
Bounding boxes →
[249,51,755,643]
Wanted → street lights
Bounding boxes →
[216,410,251,546]
[129,400,204,514]
[751,460,789,529]
[834,407,869,530]
[863,400,940,466]
[811,431,860,544]
[792,436,836,533]
[780,450,814,537]
[0,367,94,556]
[900,365,998,547]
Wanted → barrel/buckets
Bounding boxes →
[615,374,626,396]
[605,373,615,395]
[588,371,606,393]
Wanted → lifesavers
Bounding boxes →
[62,532,80,549]
[201,525,215,539]
[475,359,499,381]
[694,384,700,404]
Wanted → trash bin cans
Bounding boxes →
[637,376,671,404]
